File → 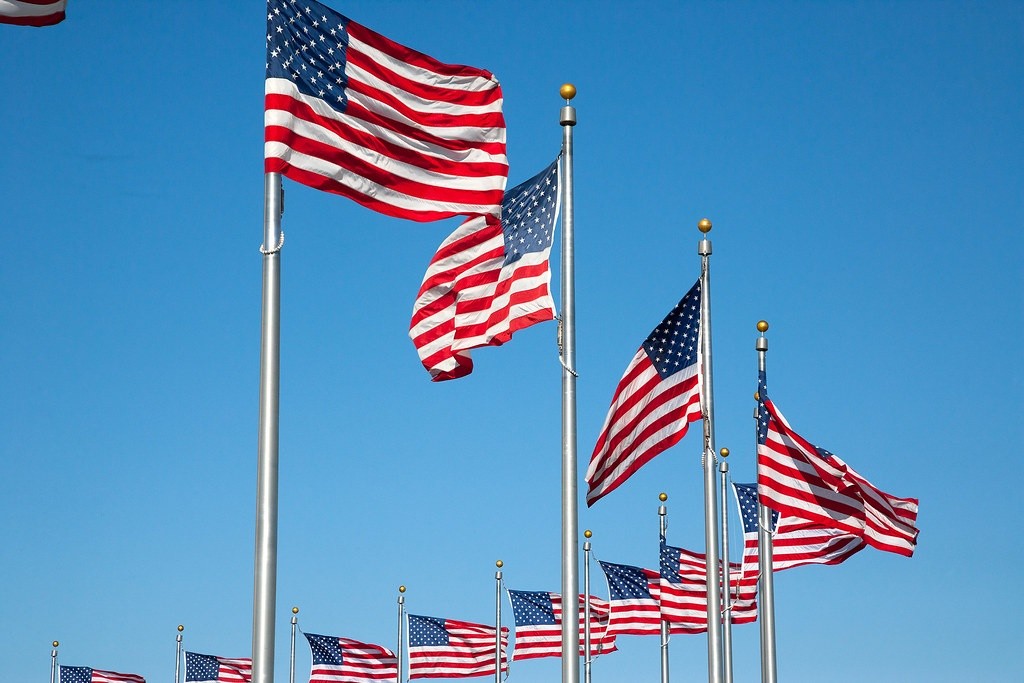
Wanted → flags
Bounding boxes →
[410,157,561,380]
[586,279,705,499]
[57,375,921,682]
[265,1,508,222]
